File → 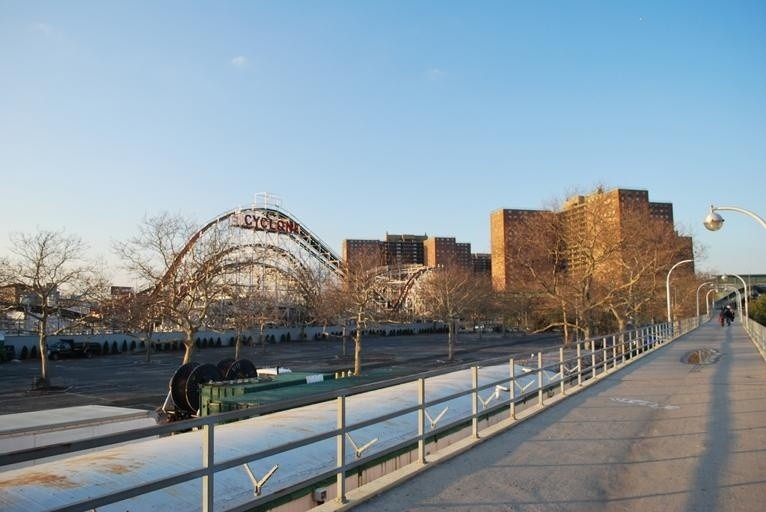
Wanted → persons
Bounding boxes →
[718,303,735,327]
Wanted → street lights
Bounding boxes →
[665,258,703,321]
[696,272,749,329]
[702,203,766,233]
